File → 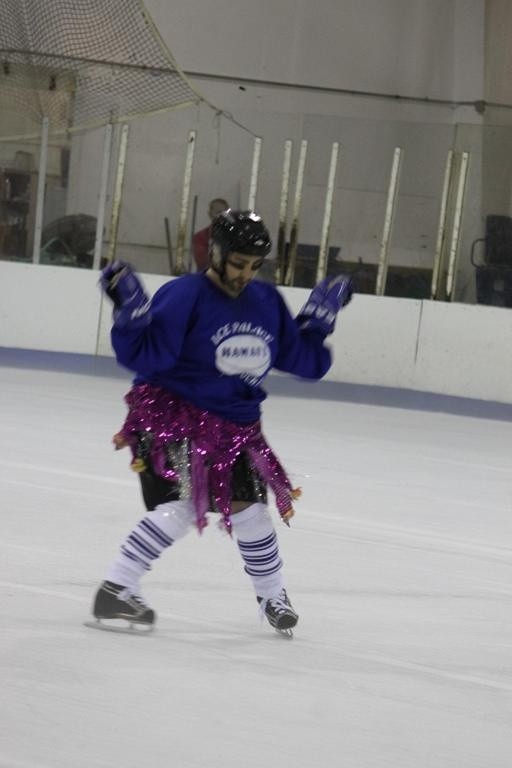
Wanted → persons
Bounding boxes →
[192,199,229,275]
[84,209,354,638]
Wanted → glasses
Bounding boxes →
[225,260,265,271]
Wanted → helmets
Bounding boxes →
[208,207,271,257]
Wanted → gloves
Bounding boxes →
[293,271,354,336]
[98,260,153,331]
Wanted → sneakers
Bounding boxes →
[257,587,298,630]
[94,580,154,626]
